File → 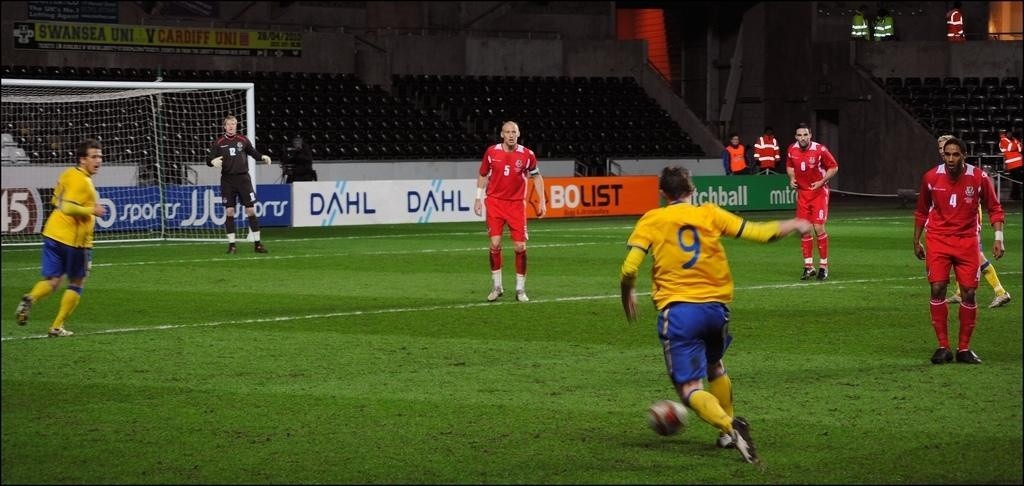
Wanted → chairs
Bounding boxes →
[875,77,1024,176]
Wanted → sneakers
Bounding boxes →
[731,416,757,463]
[988,292,1012,310]
[717,431,736,449]
[16,293,30,325]
[816,268,829,280]
[932,347,953,364]
[956,350,982,365]
[945,292,963,304]
[516,290,529,302]
[800,267,817,280]
[47,327,74,337]
[487,286,504,301]
[225,244,267,252]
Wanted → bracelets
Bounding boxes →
[993,230,1005,243]
[474,187,484,203]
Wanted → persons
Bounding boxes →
[785,123,838,280]
[472,120,547,303]
[13,136,109,338]
[204,114,273,255]
[997,125,1023,201]
[282,133,314,182]
[753,125,780,174]
[944,0,967,42]
[619,164,815,467]
[848,3,872,41]
[935,133,1012,310]
[721,133,750,175]
[909,138,1006,367]
[870,6,896,42]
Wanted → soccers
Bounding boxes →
[648,400,688,435]
